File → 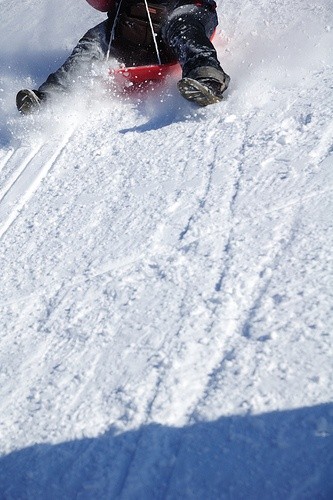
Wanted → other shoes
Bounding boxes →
[15,88,46,117]
[177,77,224,107]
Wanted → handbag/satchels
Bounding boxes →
[117,1,170,54]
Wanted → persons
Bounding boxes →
[16,0,231,118]
